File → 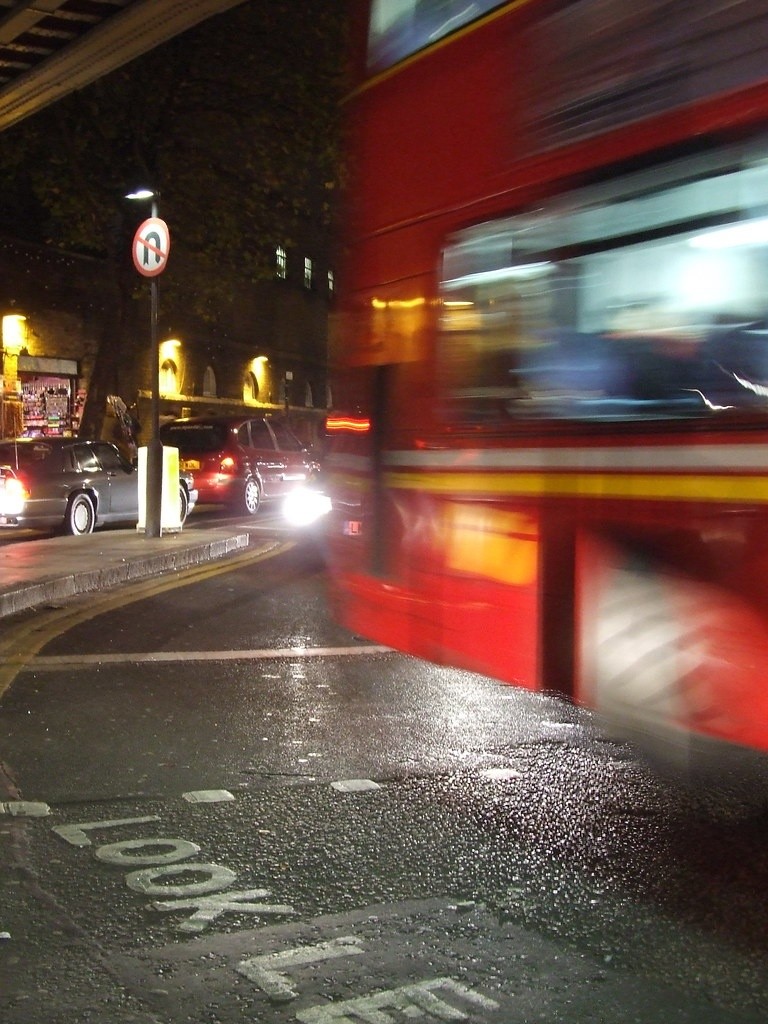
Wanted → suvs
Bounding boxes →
[161,408,324,518]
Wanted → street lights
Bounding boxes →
[127,189,162,539]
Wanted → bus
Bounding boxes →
[324,1,767,754]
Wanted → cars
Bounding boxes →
[0,434,199,541]
